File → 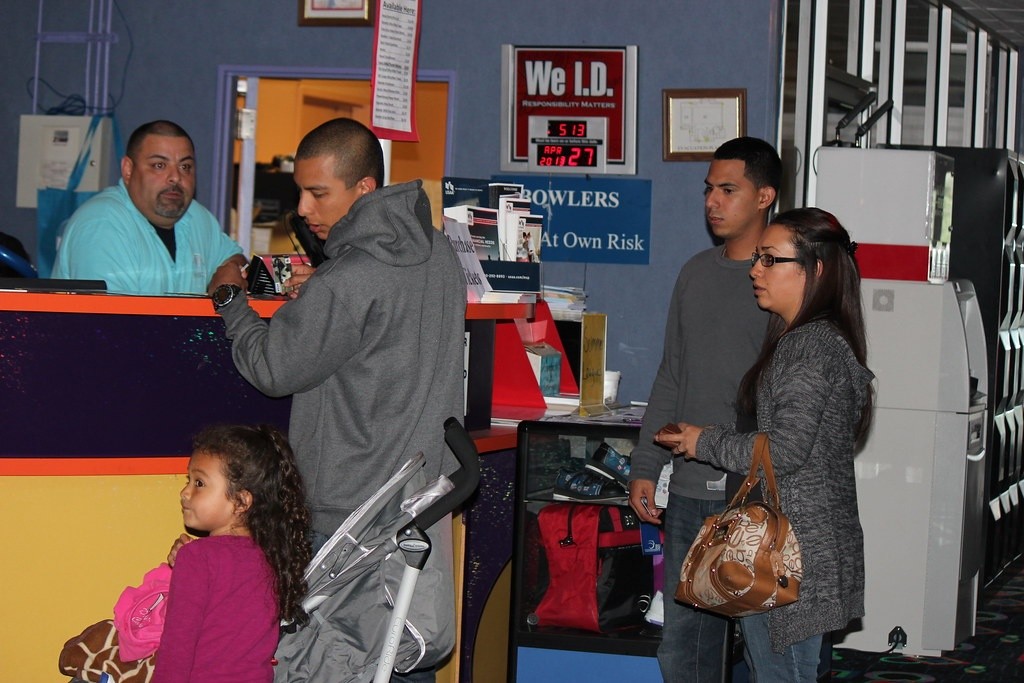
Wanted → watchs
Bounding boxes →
[211,283,242,311]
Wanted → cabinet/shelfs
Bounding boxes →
[507,412,674,683]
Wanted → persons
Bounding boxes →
[153,423,312,683]
[204,117,466,683]
[49,119,250,299]
[629,135,782,683]
[653,207,875,683]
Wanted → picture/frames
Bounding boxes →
[661,88,747,162]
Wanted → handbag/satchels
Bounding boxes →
[526,500,655,635]
[674,432,802,618]
[33,111,123,280]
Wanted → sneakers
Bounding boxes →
[553,466,630,501]
[586,441,633,492]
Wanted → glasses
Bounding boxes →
[751,251,808,268]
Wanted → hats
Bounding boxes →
[114,562,173,662]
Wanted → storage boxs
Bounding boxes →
[523,341,562,398]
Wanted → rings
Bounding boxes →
[676,446,681,453]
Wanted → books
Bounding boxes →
[440,180,588,428]
